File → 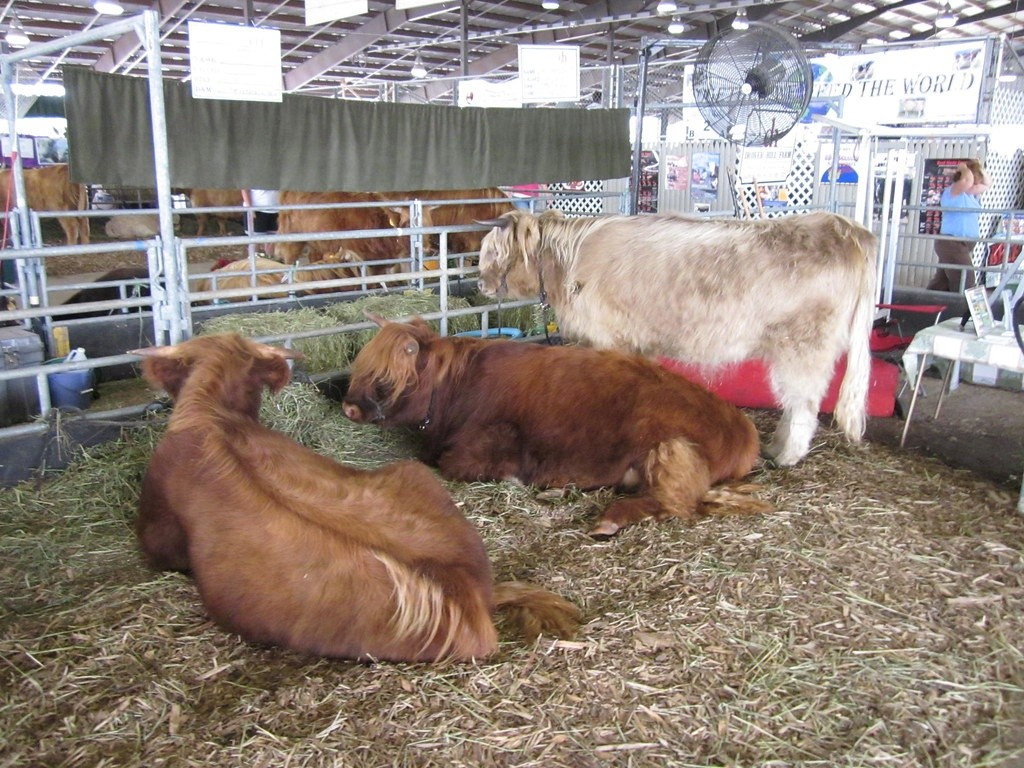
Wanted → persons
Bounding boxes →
[926,159,990,293]
[241,189,280,258]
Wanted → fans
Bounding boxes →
[691,19,814,148]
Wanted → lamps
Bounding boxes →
[731,8,749,30]
[93,0,125,16]
[668,14,684,34]
[935,3,957,28]
[656,0,678,12]
[541,0,560,10]
[410,53,427,78]
[5,9,30,46]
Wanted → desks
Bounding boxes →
[897,316,1024,448]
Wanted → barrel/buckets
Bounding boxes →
[43,357,94,411]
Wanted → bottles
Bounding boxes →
[64,347,89,372]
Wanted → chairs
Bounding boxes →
[869,304,946,398]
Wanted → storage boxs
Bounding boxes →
[0,326,45,429]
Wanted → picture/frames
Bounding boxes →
[959,284,996,337]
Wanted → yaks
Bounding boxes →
[172,188,243,237]
[342,307,760,542]
[473,209,880,470]
[0,166,91,245]
[105,213,180,239]
[373,188,519,285]
[194,246,374,304]
[51,268,167,321]
[124,334,585,666]
[277,191,442,287]
